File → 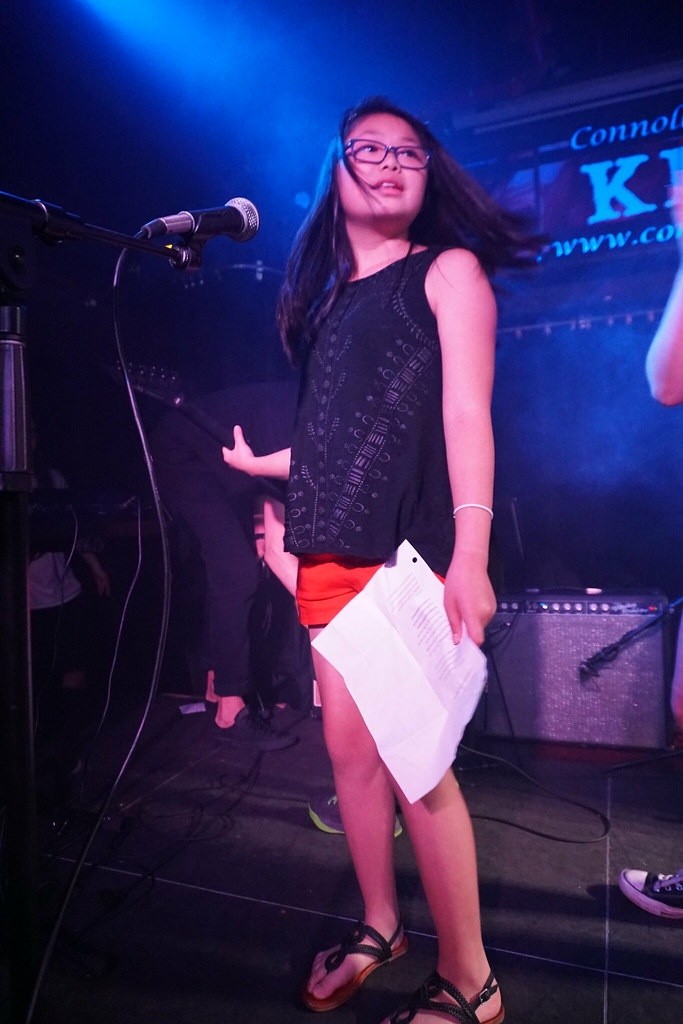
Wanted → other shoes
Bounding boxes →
[203,698,296,750]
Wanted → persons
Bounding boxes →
[28,540,109,630]
[618,183,683,916]
[220,420,402,838]
[277,99,551,1024]
[149,379,297,751]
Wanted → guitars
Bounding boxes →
[114,360,292,503]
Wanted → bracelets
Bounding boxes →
[453,504,495,520]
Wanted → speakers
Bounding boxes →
[467,588,676,750]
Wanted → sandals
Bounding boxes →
[302,910,409,1012]
[378,968,505,1024]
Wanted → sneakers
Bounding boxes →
[618,868,683,918]
[309,794,403,839]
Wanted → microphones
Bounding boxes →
[140,196,260,244]
[578,660,590,674]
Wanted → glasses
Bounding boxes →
[334,138,432,170]
